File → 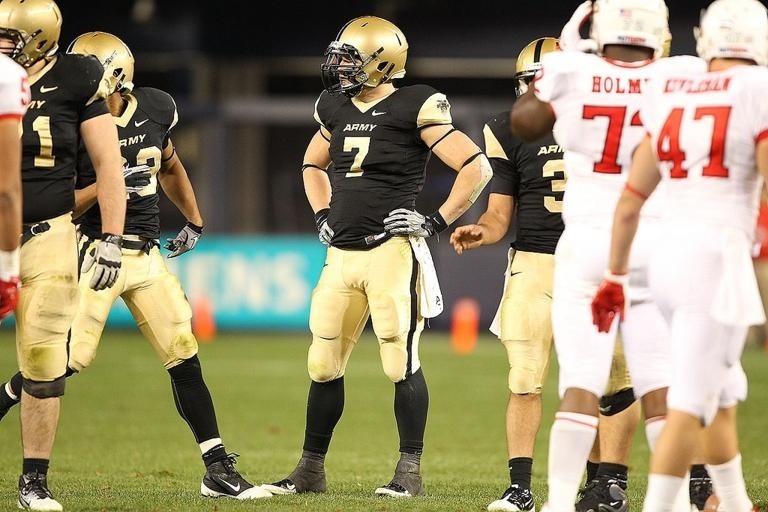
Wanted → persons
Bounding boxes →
[450,1,768,511]
[0,31,274,502]
[1,0,130,511]
[257,15,496,500]
[0,53,35,323]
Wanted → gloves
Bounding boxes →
[163,221,203,259]
[81,233,123,291]
[558,1,599,54]
[384,208,434,238]
[592,271,630,334]
[315,216,337,247]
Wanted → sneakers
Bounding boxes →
[486,482,536,511]
[261,470,327,496]
[198,459,274,500]
[574,476,723,512]
[17,469,64,512]
[375,473,423,499]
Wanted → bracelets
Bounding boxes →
[426,208,448,232]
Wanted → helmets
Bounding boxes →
[589,0,672,59]
[320,16,409,98]
[513,37,559,97]
[66,32,136,97]
[694,1,768,64]
[0,0,63,70]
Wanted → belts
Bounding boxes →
[21,220,50,246]
